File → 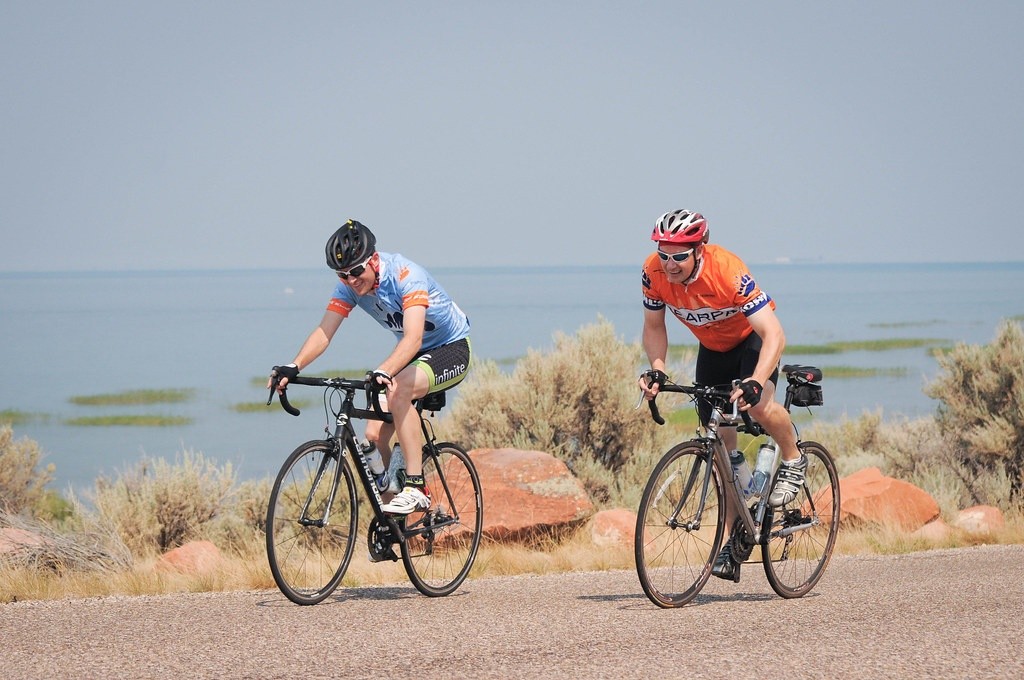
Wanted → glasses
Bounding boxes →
[656,245,694,263]
[335,256,372,280]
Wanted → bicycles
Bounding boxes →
[633,364,841,609]
[265,364,485,606]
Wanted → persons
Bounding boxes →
[266,219,474,514]
[638,209,808,582]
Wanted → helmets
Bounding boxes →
[324,219,376,275]
[651,209,709,243]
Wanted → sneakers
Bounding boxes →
[368,522,393,562]
[768,447,809,507]
[711,538,735,581]
[380,486,432,514]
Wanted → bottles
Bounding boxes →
[754,438,775,496]
[729,450,756,499]
[386,443,405,493]
[361,439,388,492]
